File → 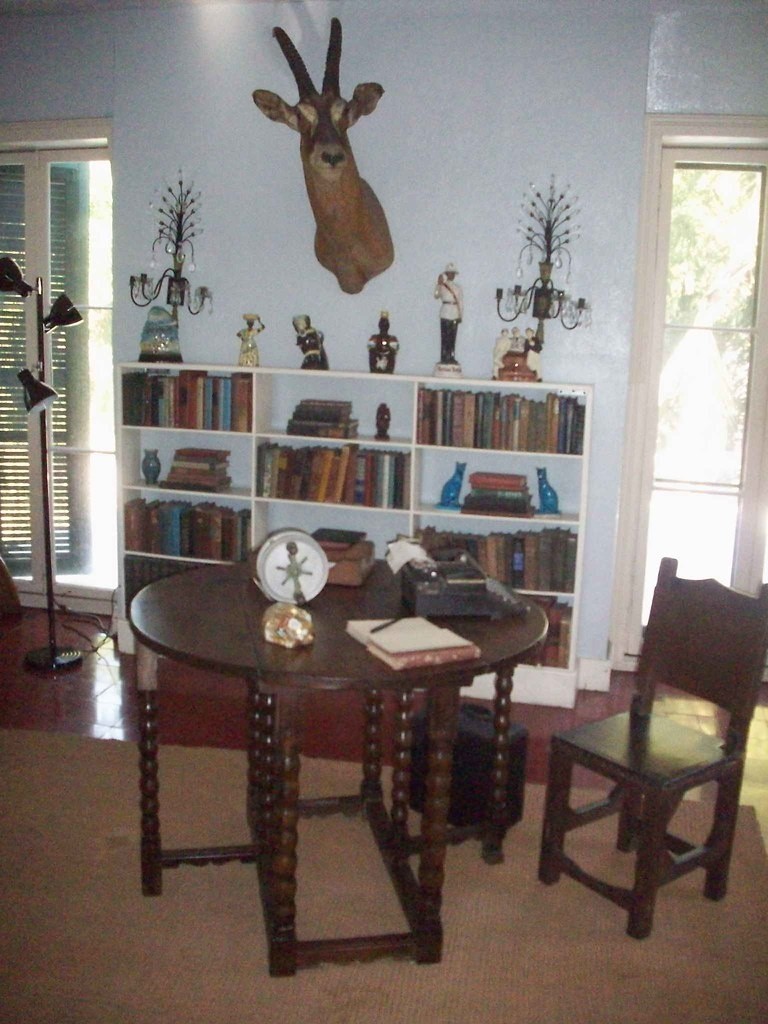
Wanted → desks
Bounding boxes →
[131,560,550,981]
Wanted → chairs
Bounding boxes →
[539,558,768,938]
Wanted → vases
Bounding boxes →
[142,449,161,485]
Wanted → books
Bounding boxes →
[521,596,572,668]
[258,442,411,510]
[160,448,232,492]
[311,529,375,588]
[346,615,482,669]
[124,499,251,562]
[286,400,356,437]
[124,556,200,620]
[417,389,585,454]
[416,527,578,593]
[460,473,534,517]
[123,369,253,433]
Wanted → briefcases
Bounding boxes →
[409,703,528,830]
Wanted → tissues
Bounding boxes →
[383,537,493,620]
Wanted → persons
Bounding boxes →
[368,317,400,373]
[292,315,328,369]
[492,327,542,383]
[434,262,464,365]
[236,317,264,367]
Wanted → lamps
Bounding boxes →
[496,174,587,341]
[0,255,83,675]
[128,168,213,327]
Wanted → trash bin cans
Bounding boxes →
[408,700,529,830]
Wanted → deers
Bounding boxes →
[251,16,396,295]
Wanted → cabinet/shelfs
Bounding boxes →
[116,362,595,710]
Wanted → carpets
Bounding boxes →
[0,728,768,1024]
